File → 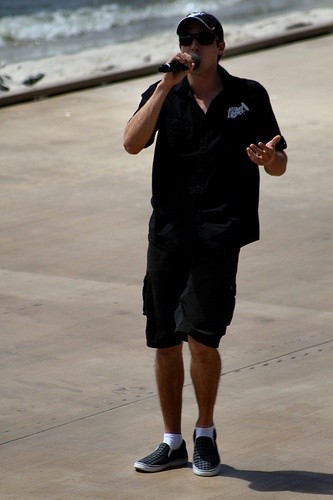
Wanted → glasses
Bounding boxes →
[178,30,218,45]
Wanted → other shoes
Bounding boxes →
[193,427,221,476]
[133,440,187,472]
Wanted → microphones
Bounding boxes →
[158,51,200,72]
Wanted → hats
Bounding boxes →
[176,11,224,40]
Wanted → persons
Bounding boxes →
[122,11,288,477]
